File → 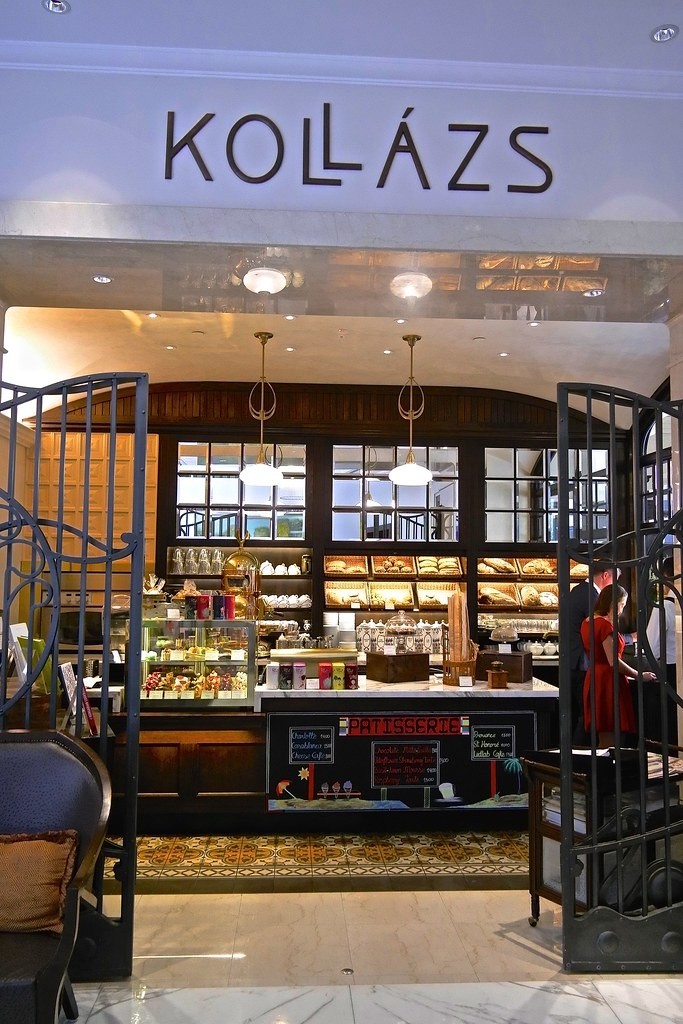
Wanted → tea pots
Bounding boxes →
[259,559,301,576]
[517,639,559,656]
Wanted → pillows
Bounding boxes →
[0,829,80,934]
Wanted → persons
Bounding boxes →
[646,555,679,758]
[581,584,657,749]
[570,558,622,749]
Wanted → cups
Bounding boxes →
[172,548,224,575]
[301,554,312,575]
[185,594,235,620]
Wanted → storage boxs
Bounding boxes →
[476,649,532,684]
[365,652,429,683]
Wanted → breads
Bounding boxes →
[325,592,440,606]
[419,556,460,574]
[325,560,365,573]
[478,558,589,607]
[375,556,413,573]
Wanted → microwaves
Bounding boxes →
[40,606,130,653]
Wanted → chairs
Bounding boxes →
[0,729,112,1024]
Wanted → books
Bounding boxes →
[9,622,103,735]
[542,787,679,834]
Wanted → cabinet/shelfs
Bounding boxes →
[164,538,612,666]
[520,739,683,927]
[264,247,610,307]
[125,619,256,710]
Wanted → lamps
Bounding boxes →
[356,446,382,515]
[238,333,284,486]
[388,335,433,486]
[243,267,287,314]
[391,271,433,317]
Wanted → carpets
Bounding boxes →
[85,831,530,894]
